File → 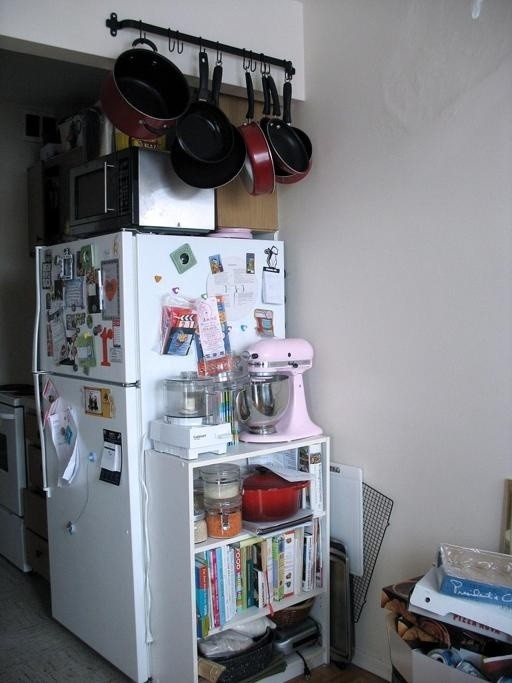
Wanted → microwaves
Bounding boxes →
[66,146,216,238]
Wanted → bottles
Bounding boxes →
[192,463,243,544]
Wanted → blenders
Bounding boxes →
[236,337,327,443]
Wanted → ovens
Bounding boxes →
[0,401,37,572]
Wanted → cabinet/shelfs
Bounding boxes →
[139,437,332,683]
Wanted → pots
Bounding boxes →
[101,34,313,196]
[241,463,310,523]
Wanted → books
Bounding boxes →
[194,523,322,641]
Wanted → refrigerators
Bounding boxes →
[32,231,290,682]
